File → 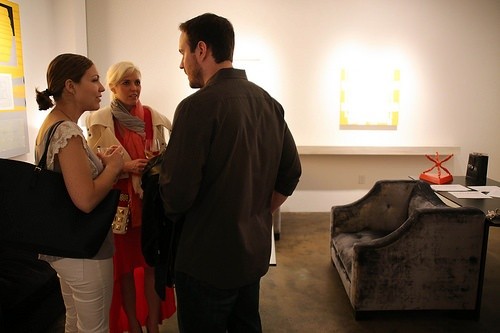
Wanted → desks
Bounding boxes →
[437,175,500,320]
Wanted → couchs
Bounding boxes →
[330,179,485,320]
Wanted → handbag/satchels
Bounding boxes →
[0,120,122,259]
[111,206,131,234]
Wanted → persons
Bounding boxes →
[32,53,127,332]
[87,60,178,333]
[137,12,305,333]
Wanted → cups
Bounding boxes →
[145,137,162,158]
[97,147,116,165]
